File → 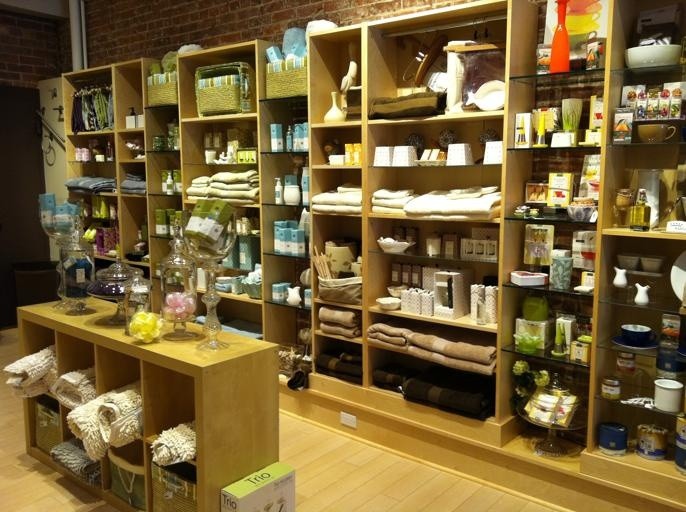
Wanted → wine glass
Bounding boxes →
[39,197,83,310]
[180,211,236,350]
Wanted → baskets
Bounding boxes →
[145,72,177,107]
[151,462,196,511]
[264,56,308,101]
[192,61,257,117]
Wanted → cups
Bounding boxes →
[621,325,656,345]
[638,123,676,143]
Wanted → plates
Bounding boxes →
[613,335,658,349]
[376,237,417,312]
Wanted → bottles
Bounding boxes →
[59,235,198,340]
[613,168,660,231]
[324,92,346,122]
[550,0,570,75]
[602,352,636,402]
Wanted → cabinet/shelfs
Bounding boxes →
[497,0,686,509]
[61,0,509,480]
[17,297,279,512]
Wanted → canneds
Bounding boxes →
[152,134,165,151]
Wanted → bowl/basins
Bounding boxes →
[624,45,681,70]
